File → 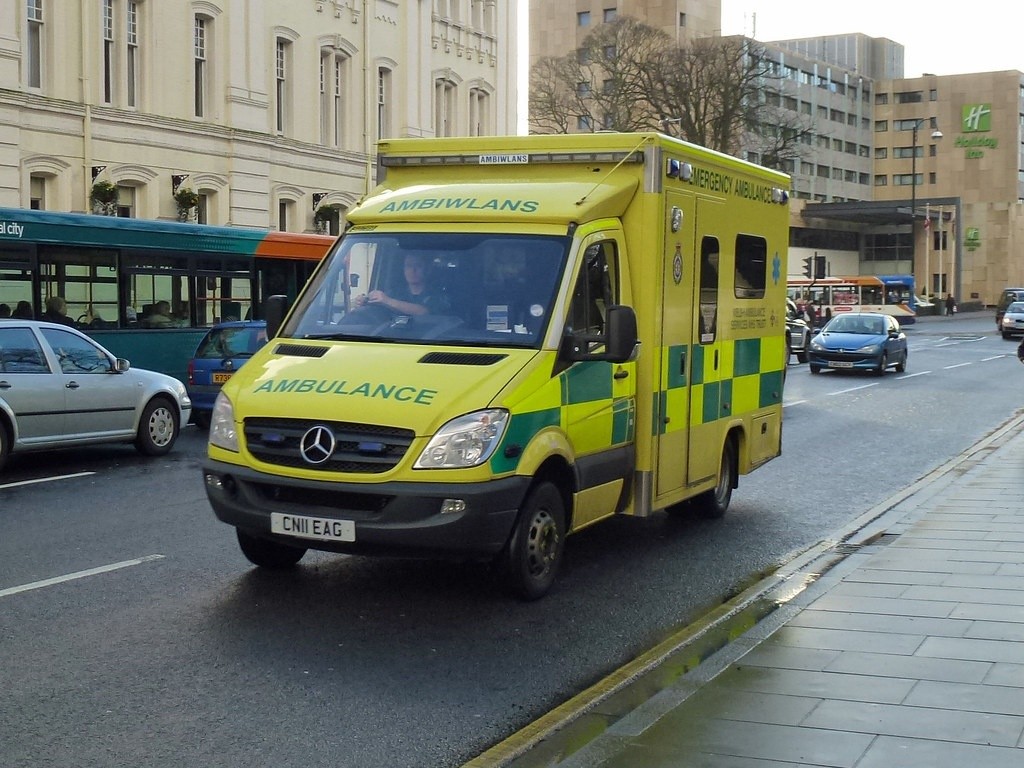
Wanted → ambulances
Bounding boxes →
[201,130,792,604]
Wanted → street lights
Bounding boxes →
[911,118,943,320]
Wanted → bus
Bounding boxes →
[1,204,360,404]
[784,273,916,329]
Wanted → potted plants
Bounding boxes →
[314,205,340,235]
[89,180,120,217]
[173,187,201,224]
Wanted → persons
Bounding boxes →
[0,296,178,329]
[364,249,464,316]
[945,293,957,316]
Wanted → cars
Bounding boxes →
[783,295,812,363]
[187,322,269,433]
[0,317,193,468]
[808,312,909,376]
[995,287,1024,339]
